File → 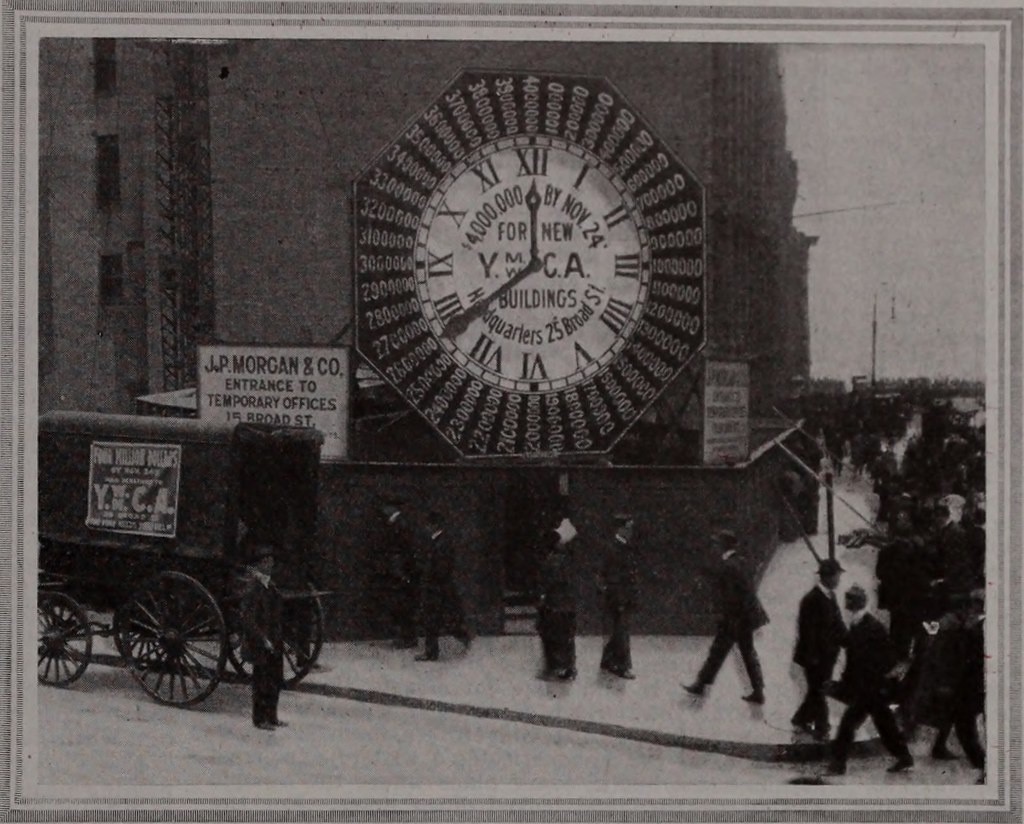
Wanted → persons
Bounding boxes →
[381,492,426,649]
[538,530,578,681]
[597,512,638,679]
[806,374,985,770]
[240,546,291,732]
[788,559,849,742]
[414,510,472,662]
[681,530,769,704]
[818,585,914,775]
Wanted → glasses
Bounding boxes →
[844,592,858,600]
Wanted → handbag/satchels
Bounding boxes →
[744,591,771,629]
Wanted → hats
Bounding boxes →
[817,560,845,576]
[254,549,272,556]
[711,532,740,547]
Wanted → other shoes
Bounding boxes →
[741,692,764,703]
[460,628,474,651]
[603,659,620,671]
[888,755,915,772]
[618,669,636,679]
[258,721,275,729]
[791,720,830,738]
[417,644,441,660]
[934,746,962,760]
[274,719,289,727]
[544,668,579,678]
[821,764,847,774]
[679,683,706,696]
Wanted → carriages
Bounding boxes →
[39,542,330,714]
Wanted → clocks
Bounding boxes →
[351,69,712,462]
